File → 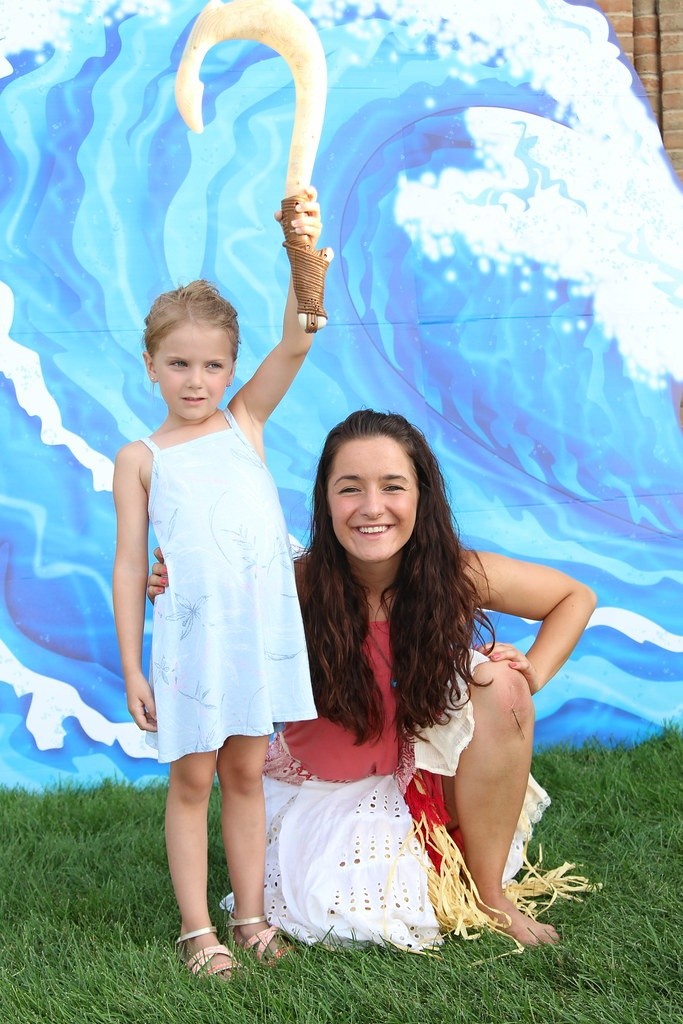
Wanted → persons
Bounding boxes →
[147,410,597,950]
[112,187,322,985]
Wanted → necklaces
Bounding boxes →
[371,619,398,690]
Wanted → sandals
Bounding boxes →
[226,912,295,967]
[178,926,242,978]
